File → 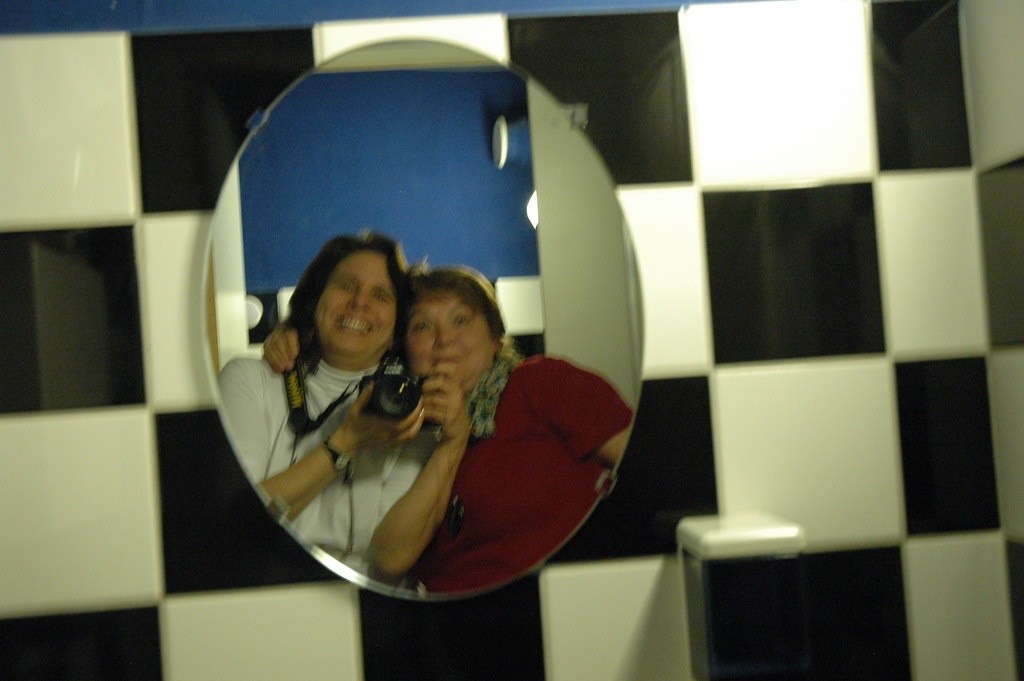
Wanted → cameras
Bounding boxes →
[359,356,440,429]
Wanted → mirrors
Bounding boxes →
[197,36,645,607]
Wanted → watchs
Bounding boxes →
[323,436,350,470]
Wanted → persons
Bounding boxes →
[264,265,632,594]
[219,231,470,581]
